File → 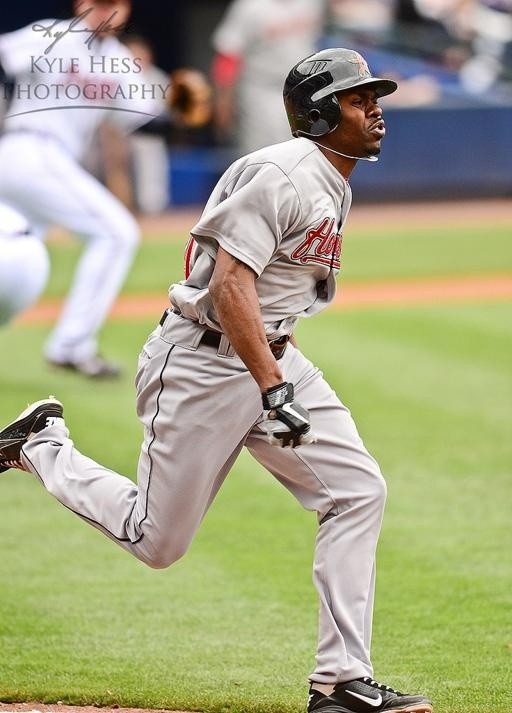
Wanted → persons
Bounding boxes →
[1,44,434,713]
[2,1,512,381]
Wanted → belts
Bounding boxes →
[161,308,289,360]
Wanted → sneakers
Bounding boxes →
[309,674,434,713]
[48,350,118,381]
[0,399,64,473]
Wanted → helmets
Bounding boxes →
[283,47,397,138]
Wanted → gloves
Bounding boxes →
[252,381,315,448]
